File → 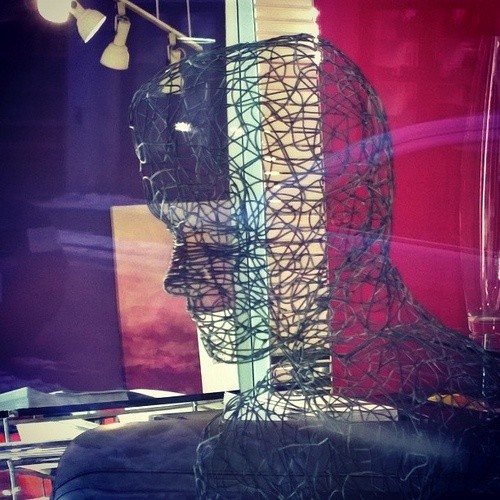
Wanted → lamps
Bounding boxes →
[99,15,132,71]
[36,0,107,44]
[159,45,185,95]
